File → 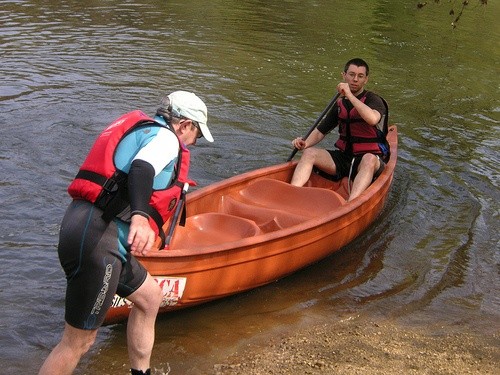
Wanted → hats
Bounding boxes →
[160,90,214,142]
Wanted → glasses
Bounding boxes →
[192,122,203,138]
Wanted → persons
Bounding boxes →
[36,91,214,375]
[291,57,391,201]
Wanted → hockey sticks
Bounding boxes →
[164,180,190,250]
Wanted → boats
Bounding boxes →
[100,124,398,327]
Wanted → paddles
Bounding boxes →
[288,90,341,160]
